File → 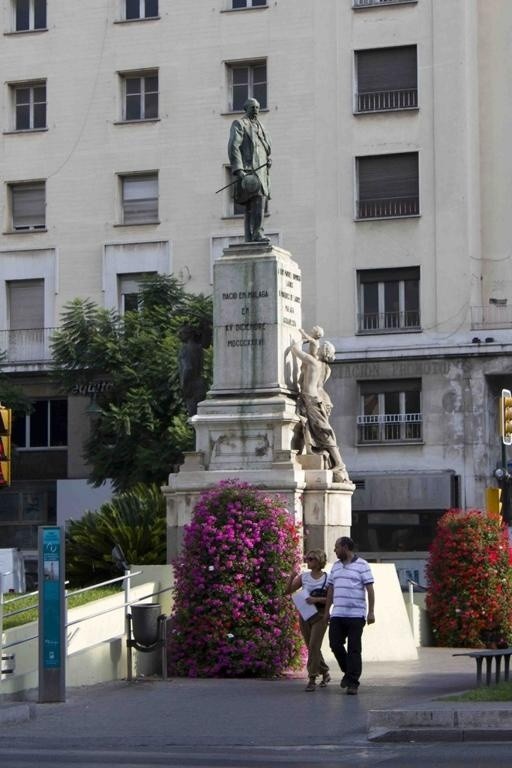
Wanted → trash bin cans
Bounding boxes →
[125,603,168,681]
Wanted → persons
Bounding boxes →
[292,338,345,472]
[286,548,331,691]
[229,97,273,241]
[297,326,323,385]
[324,537,375,694]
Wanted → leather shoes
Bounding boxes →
[341,674,357,694]
[306,673,330,691]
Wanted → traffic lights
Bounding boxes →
[0,405,10,486]
[486,487,504,527]
[499,395,512,437]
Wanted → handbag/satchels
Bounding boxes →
[310,589,327,607]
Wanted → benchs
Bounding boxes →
[451,645,512,688]
